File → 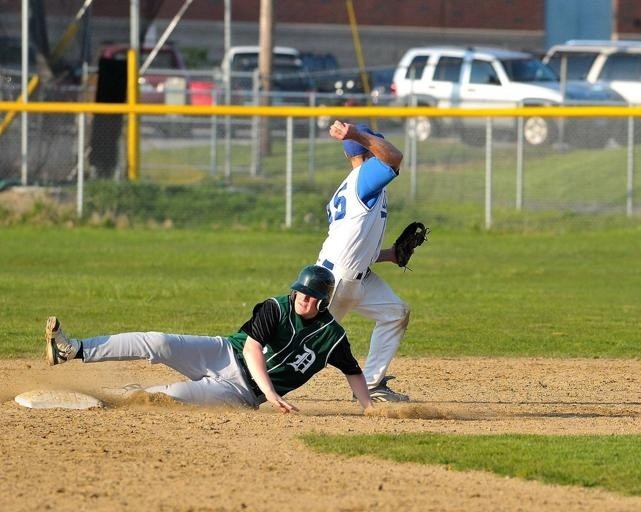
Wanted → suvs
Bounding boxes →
[388,43,628,149]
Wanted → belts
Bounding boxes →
[322,259,371,280]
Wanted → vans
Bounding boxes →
[219,44,316,140]
[536,37,641,142]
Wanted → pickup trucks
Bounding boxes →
[45,41,221,141]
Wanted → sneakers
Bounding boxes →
[46,317,81,365]
[353,376,409,402]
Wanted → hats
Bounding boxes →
[342,125,384,158]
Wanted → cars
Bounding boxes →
[313,64,396,132]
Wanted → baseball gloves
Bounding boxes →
[394,221,431,272]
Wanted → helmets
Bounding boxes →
[291,266,335,312]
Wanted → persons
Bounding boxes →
[314,120,426,402]
[44,265,373,415]
